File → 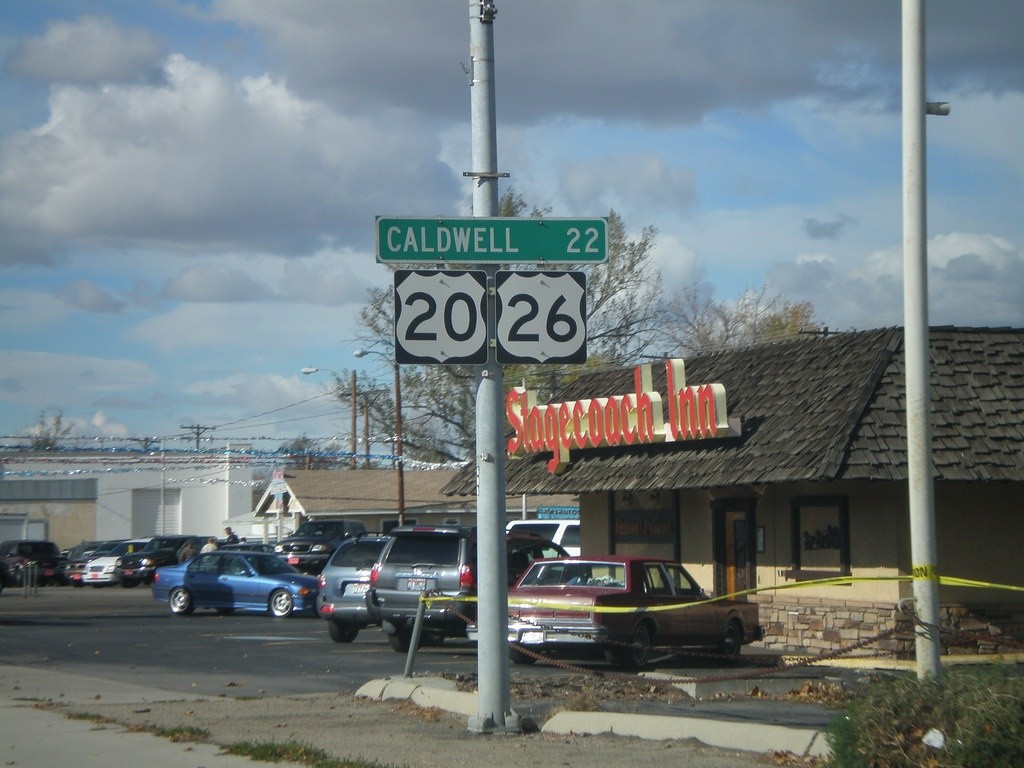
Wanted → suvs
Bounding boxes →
[272,520,367,576]
[365,522,571,652]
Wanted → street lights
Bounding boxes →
[354,349,406,528]
[300,367,360,470]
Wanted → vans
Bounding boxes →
[505,520,581,569]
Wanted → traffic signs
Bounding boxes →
[372,213,611,268]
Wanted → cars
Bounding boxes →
[149,548,319,619]
[464,553,766,670]
[0,515,273,587]
[313,531,392,641]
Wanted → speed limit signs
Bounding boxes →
[492,267,591,366]
[390,268,490,367]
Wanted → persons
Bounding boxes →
[181,542,198,561]
[225,527,248,551]
[199,538,218,562]
